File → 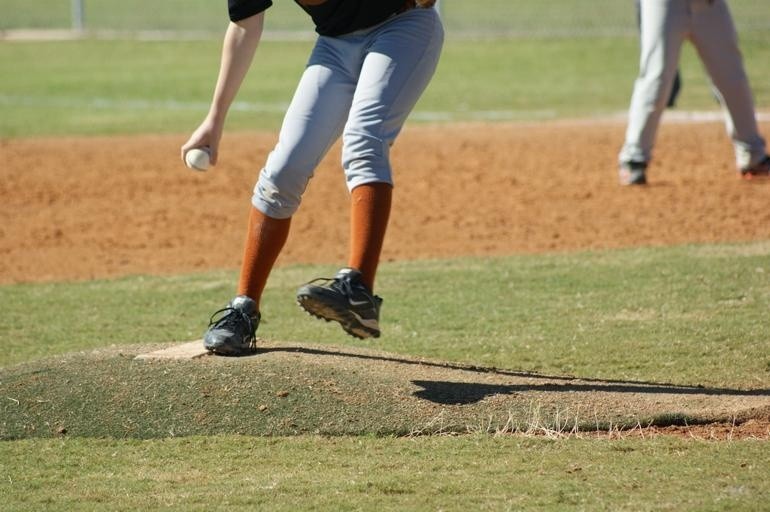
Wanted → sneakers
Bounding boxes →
[204,296,261,357]
[619,164,645,184]
[736,154,769,181]
[296,267,383,340]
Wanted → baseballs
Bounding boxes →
[186,149,210,173]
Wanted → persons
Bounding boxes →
[615,0,769,185]
[178,0,446,356]
